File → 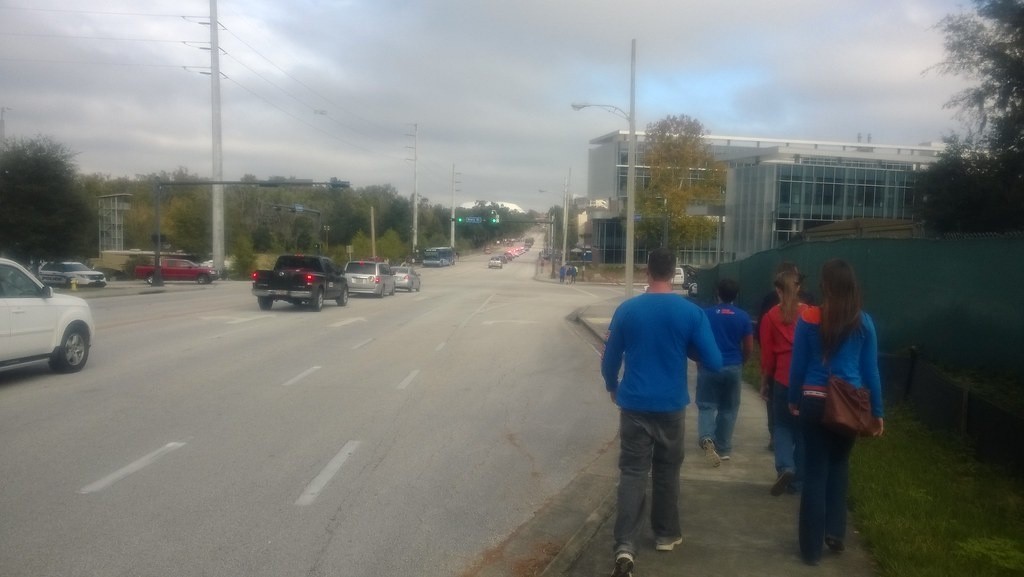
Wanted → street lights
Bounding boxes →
[570,100,636,296]
[538,189,568,266]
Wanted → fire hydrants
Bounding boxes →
[70,276,78,289]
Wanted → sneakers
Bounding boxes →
[656,536,682,550]
[612,552,634,577]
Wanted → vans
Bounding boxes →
[344,261,396,298]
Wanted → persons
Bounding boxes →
[601,249,724,577]
[560,264,579,285]
[758,260,816,496]
[789,261,885,566]
[695,279,754,467]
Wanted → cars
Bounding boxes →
[38,262,106,288]
[508,238,534,258]
[497,255,507,264]
[390,267,421,292]
[504,253,512,260]
[0,259,95,372]
[489,257,502,268]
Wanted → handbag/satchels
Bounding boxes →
[822,376,872,431]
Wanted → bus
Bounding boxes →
[423,247,453,267]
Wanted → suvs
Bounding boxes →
[671,268,685,285]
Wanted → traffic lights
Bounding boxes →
[289,208,295,212]
[487,219,496,223]
[452,218,461,221]
[274,206,281,210]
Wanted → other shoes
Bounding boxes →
[704,439,720,467]
[721,455,730,460]
[826,539,843,551]
[806,560,818,566]
[771,477,784,496]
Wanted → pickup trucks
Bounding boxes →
[252,255,349,312]
[135,258,219,284]
